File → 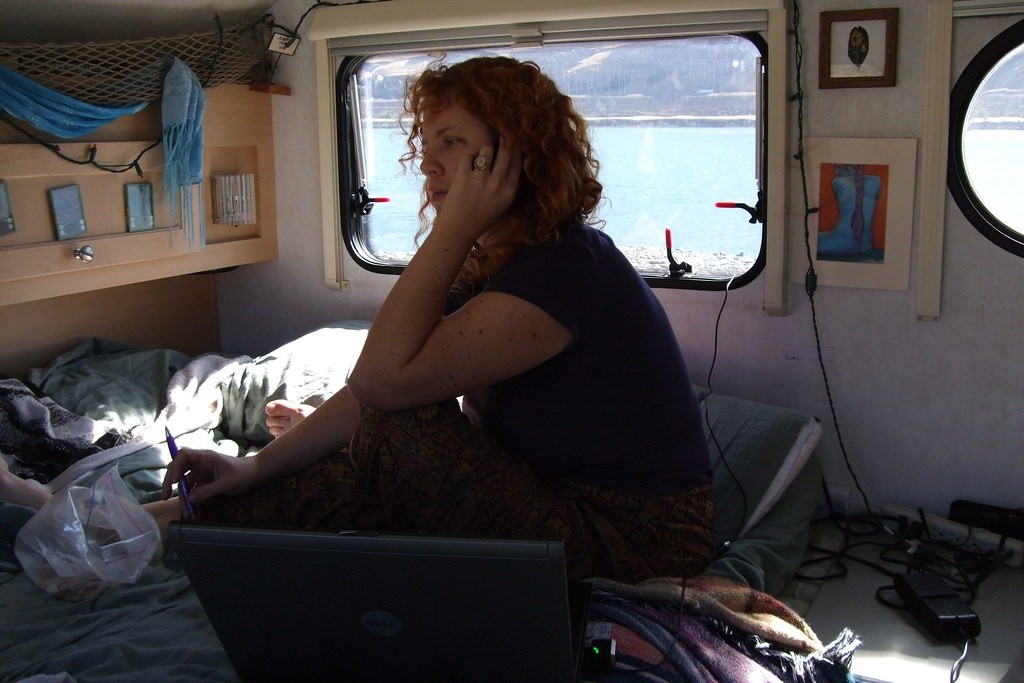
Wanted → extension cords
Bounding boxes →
[878,502,1024,568]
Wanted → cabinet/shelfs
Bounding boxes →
[0,84,278,308]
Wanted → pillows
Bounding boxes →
[219,318,823,554]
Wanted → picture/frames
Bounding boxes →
[799,136,918,291]
[819,7,899,90]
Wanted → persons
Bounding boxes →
[0,57,716,586]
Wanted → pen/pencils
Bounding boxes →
[165,428,196,521]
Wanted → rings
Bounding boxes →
[474,155,491,170]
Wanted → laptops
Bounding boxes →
[167,520,592,683]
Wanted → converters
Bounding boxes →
[893,571,981,641]
